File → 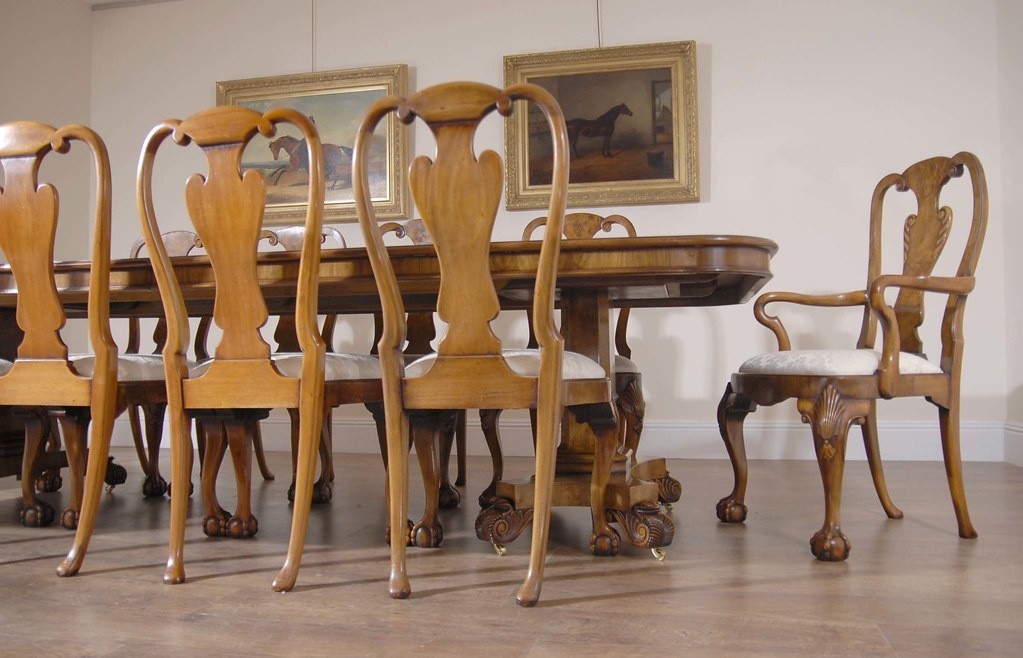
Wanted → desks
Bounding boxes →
[0,231,780,553]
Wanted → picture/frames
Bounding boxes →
[214,63,410,226]
[503,39,701,212]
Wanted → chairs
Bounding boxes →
[0,77,645,605]
[714,152,996,563]
